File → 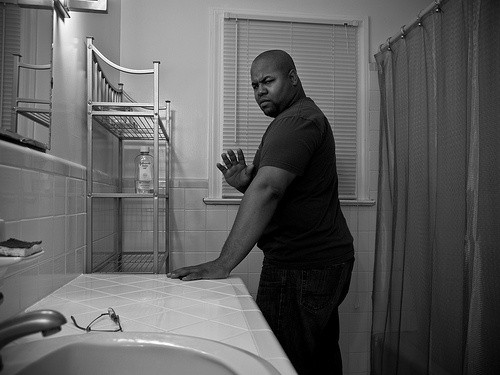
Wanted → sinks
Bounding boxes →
[1,331,284,375]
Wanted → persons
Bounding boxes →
[165,48,357,375]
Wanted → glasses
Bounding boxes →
[71,307,124,334]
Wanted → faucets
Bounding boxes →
[0,309,68,350]
